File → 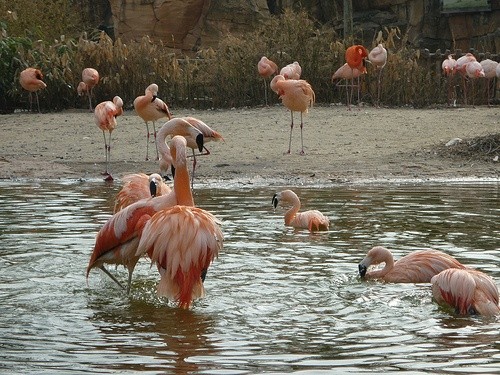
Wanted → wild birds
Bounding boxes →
[494,62,500,78]
[465,57,486,110]
[112,170,172,216]
[430,267,500,319]
[441,53,456,103]
[454,52,478,107]
[86,117,206,296]
[279,60,303,81]
[367,43,388,110]
[133,134,226,324]
[132,82,171,163]
[344,44,370,112]
[356,243,466,284]
[93,95,124,181]
[269,75,316,157]
[479,58,498,109]
[18,67,47,114]
[271,189,330,232]
[178,116,228,178]
[331,61,368,108]
[257,56,279,109]
[76,67,100,113]
